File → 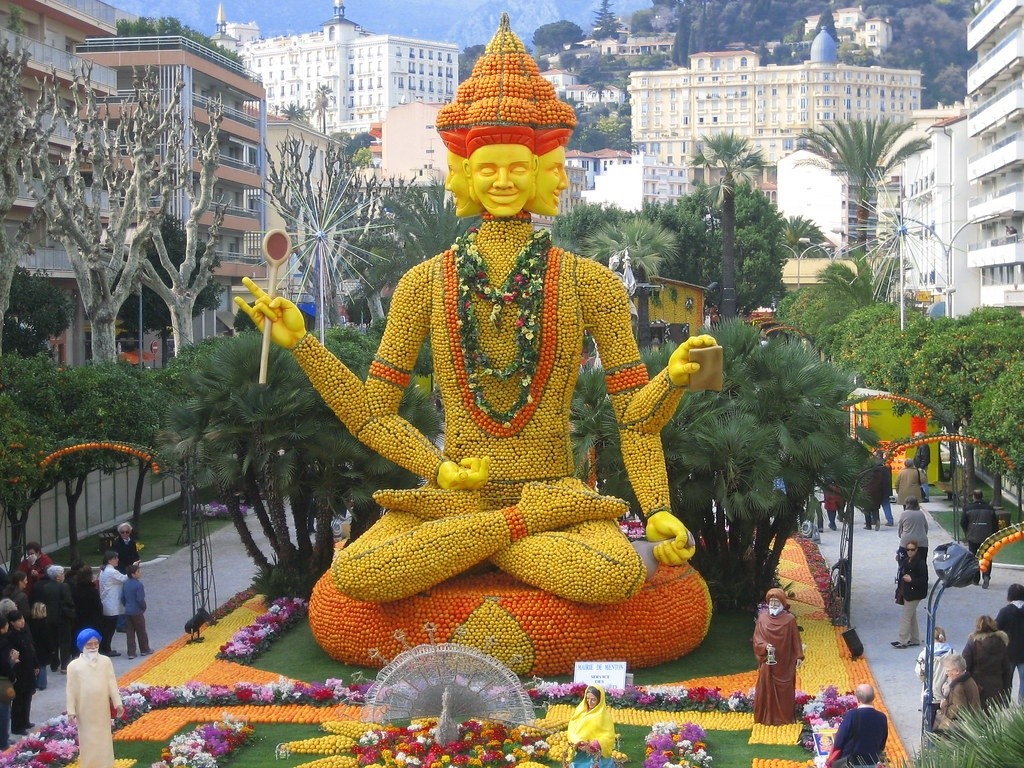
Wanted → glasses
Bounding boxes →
[906,548,917,552]
[121,531,130,535]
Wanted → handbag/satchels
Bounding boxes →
[894,588,904,604]
[916,468,926,499]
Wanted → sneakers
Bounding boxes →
[891,641,907,649]
[907,640,920,646]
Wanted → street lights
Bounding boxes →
[881,209,1002,317]
[776,241,830,288]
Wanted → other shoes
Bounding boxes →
[818,528,823,532]
[982,576,989,589]
[886,522,892,525]
[970,580,980,585]
[141,648,153,656]
[920,498,928,503]
[129,653,137,659]
[0,738,16,752]
[29,723,35,728]
[21,730,28,735]
[875,521,880,531]
[61,668,67,673]
[51,660,60,672]
[863,526,872,530]
[105,650,122,657]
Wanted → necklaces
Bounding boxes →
[446,229,550,424]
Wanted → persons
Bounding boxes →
[0,542,104,750]
[826,684,888,768]
[234,13,724,605]
[960,489,1000,589]
[895,462,926,509]
[111,523,141,633]
[898,496,929,576]
[120,565,154,659]
[891,540,928,648]
[302,464,349,535]
[807,450,894,533]
[601,256,636,334]
[915,583,1024,752]
[66,628,124,768]
[912,431,931,502]
[98,550,141,657]
[753,588,806,726]
[567,684,615,768]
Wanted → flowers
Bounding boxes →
[215,597,308,666]
[619,518,646,539]
[794,530,844,619]
[0,677,891,768]
[206,586,257,627]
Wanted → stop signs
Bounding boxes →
[151,340,159,354]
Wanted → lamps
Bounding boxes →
[842,627,864,662]
[185,608,211,642]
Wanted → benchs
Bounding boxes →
[932,467,966,501]
